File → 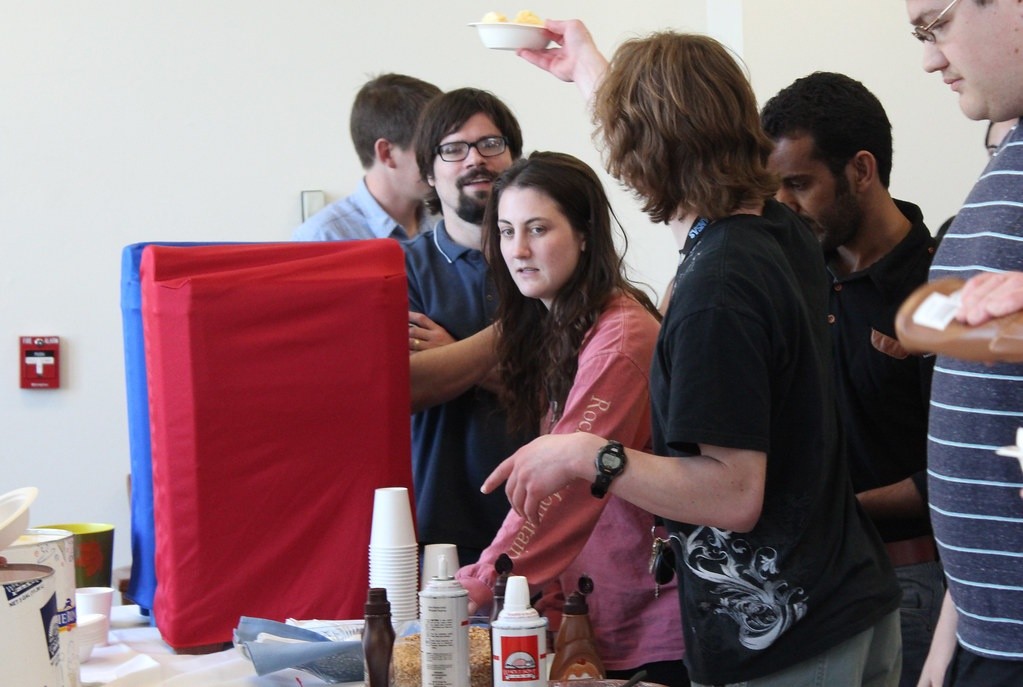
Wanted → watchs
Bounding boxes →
[589,439,627,498]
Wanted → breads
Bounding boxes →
[391,625,495,687]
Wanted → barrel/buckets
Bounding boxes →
[0,563,65,687]
[0,528,81,687]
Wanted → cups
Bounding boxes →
[76,587,113,646]
[368,486,461,634]
[37,523,115,585]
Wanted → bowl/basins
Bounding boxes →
[0,486,38,551]
[466,22,554,52]
[78,613,109,663]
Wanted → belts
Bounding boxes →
[883,540,935,568]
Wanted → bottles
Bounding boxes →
[547,575,606,681]
[491,574,550,687]
[489,552,513,687]
[417,555,472,687]
[361,587,396,687]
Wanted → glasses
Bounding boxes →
[435,135,508,162]
[911,0,957,43]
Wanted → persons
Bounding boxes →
[908,0,1023,687]
[454,150,689,686]
[757,72,947,687]
[480,18,903,687]
[985,117,1019,161]
[286,73,443,248]
[399,89,523,574]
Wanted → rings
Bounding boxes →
[414,339,420,350]
